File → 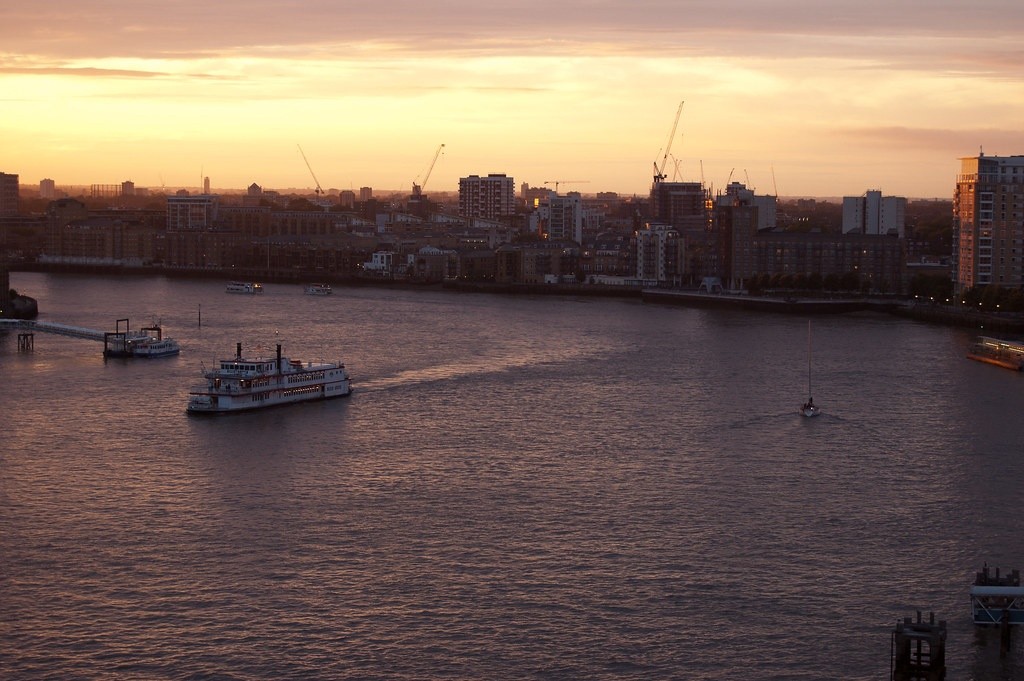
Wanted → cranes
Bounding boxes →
[649,98,779,200]
[544,179,591,197]
[297,144,325,195]
[410,144,447,198]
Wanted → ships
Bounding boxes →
[184,341,352,414]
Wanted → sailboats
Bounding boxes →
[800,320,820,418]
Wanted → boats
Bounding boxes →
[303,282,333,296]
[224,280,264,294]
[132,337,179,358]
[968,335,1024,371]
